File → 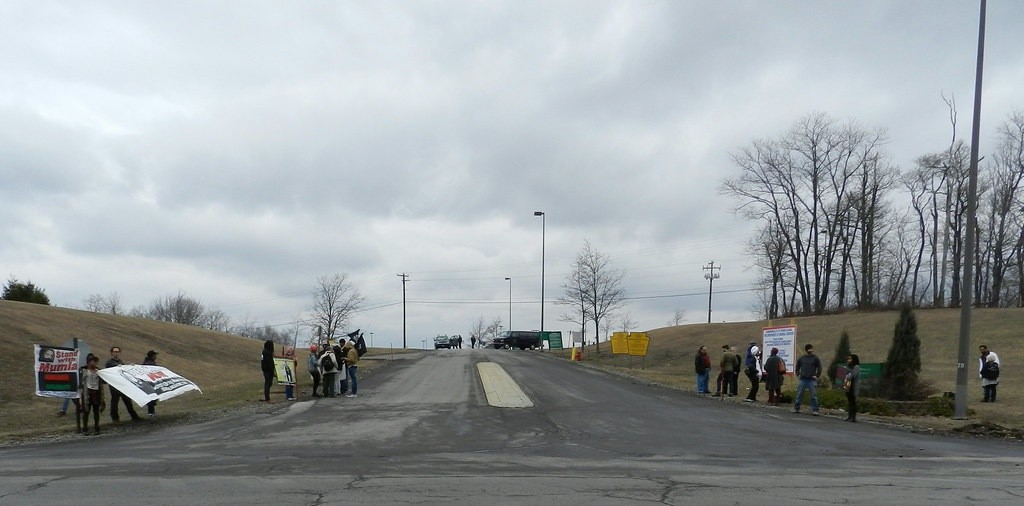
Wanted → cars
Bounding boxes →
[433,333,459,349]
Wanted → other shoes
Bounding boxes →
[94,430,100,434]
[346,394,357,398]
[287,391,335,400]
[980,399,989,403]
[745,397,755,402]
[83,430,90,436]
[844,417,856,422]
[812,411,819,415]
[792,408,799,413]
[56,411,66,417]
[991,399,996,403]
[712,392,721,396]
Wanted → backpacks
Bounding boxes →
[322,354,333,371]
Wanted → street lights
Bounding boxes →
[397,273,410,348]
[505,277,512,331]
[533,211,546,351]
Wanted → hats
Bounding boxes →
[86,353,99,362]
[147,350,159,357]
[750,340,757,344]
[310,345,317,352]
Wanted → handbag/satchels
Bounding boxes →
[778,358,786,374]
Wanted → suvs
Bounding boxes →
[491,329,544,351]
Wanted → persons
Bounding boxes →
[979,345,1000,402]
[285,346,297,400]
[844,354,860,421]
[453,336,458,349]
[308,339,358,398]
[471,335,476,349]
[711,344,741,397]
[792,344,822,415]
[261,340,274,401]
[764,348,785,403]
[144,350,159,415]
[106,347,144,420]
[458,335,462,349]
[39,347,54,363]
[695,346,712,393]
[57,352,106,436]
[745,340,762,402]
